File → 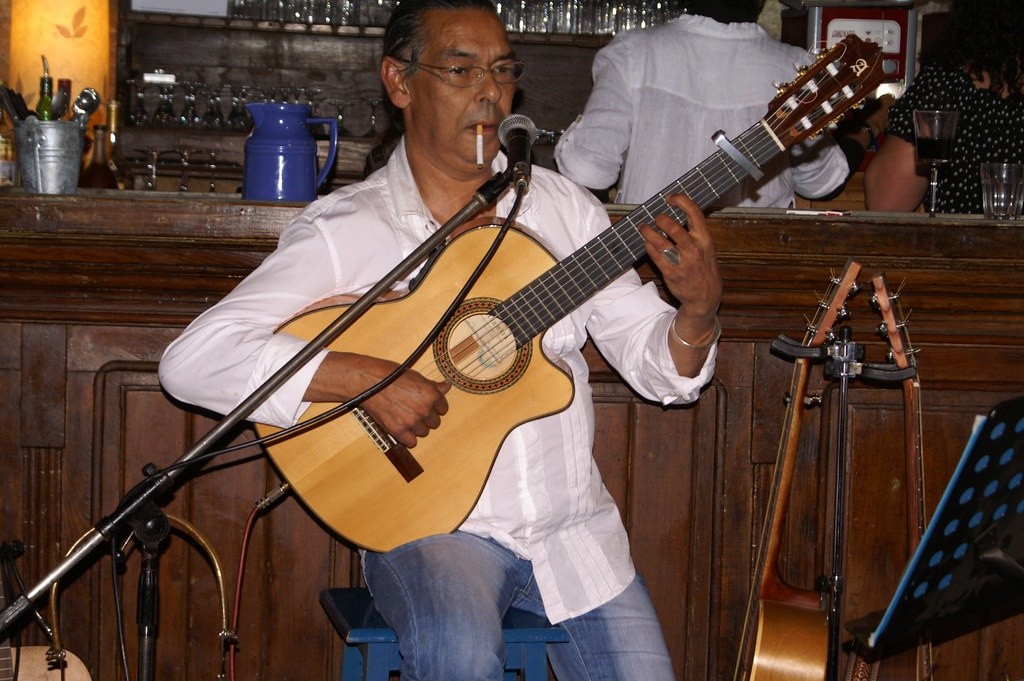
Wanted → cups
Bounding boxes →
[494,0,677,35]
[231,0,401,27]
[981,163,1024,221]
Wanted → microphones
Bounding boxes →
[498,113,537,187]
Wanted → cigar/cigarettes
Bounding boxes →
[478,125,484,169]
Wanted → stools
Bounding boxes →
[317,587,570,681]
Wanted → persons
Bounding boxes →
[864,0,1024,215]
[159,0,720,681]
[553,0,894,210]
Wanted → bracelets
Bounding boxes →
[859,122,875,147]
[670,316,722,348]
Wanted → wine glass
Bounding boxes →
[250,86,326,135]
[126,81,179,127]
[329,101,352,137]
[179,81,252,131]
[174,146,203,192]
[135,146,173,191]
[913,110,957,220]
[361,98,383,137]
[203,149,226,192]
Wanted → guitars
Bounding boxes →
[731,255,864,680]
[871,270,937,680]
[252,32,906,554]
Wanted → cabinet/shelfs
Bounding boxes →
[113,0,687,201]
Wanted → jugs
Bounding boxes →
[243,103,338,204]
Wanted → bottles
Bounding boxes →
[82,125,118,190]
[37,78,52,118]
[106,100,133,191]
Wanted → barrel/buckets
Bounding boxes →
[14,116,87,196]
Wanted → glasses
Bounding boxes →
[401,59,524,87]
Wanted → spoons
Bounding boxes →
[51,92,68,122]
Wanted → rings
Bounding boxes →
[660,248,681,265]
